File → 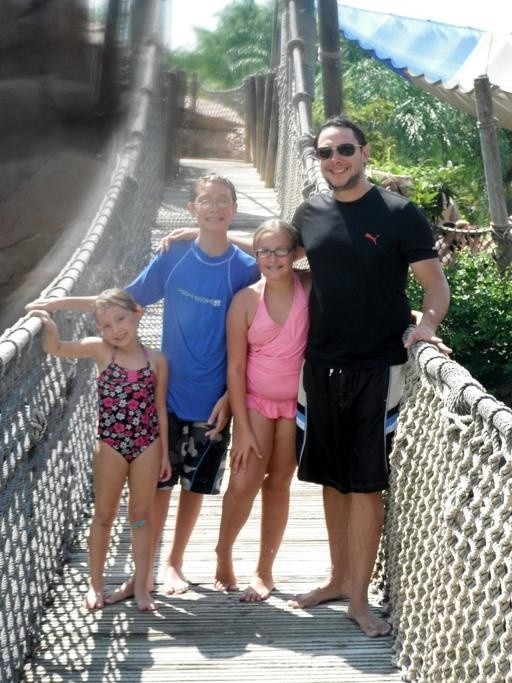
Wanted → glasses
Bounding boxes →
[314,143,364,158]
[252,243,295,257]
[191,195,235,210]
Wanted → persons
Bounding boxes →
[23,172,265,604]
[159,114,452,641]
[30,286,169,612]
[212,216,423,602]
